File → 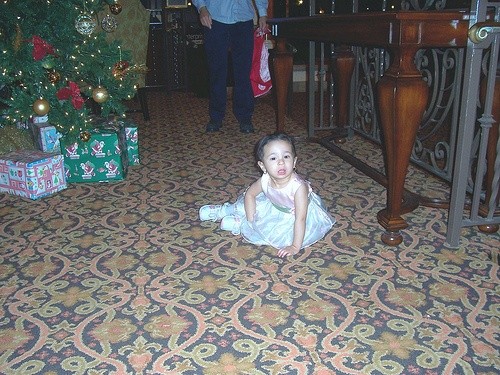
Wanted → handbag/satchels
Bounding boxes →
[250,24,273,98]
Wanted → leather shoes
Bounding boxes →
[239,121,255,134]
[207,120,223,132]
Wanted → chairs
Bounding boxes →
[77,0,151,122]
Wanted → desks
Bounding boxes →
[266,9,500,246]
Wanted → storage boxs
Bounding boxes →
[0,150,67,200]
[59,130,125,183]
[117,123,140,166]
[33,122,63,152]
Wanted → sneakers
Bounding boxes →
[199,205,226,222]
[221,216,242,231]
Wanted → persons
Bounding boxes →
[193,0,269,132]
[199,132,337,259]
